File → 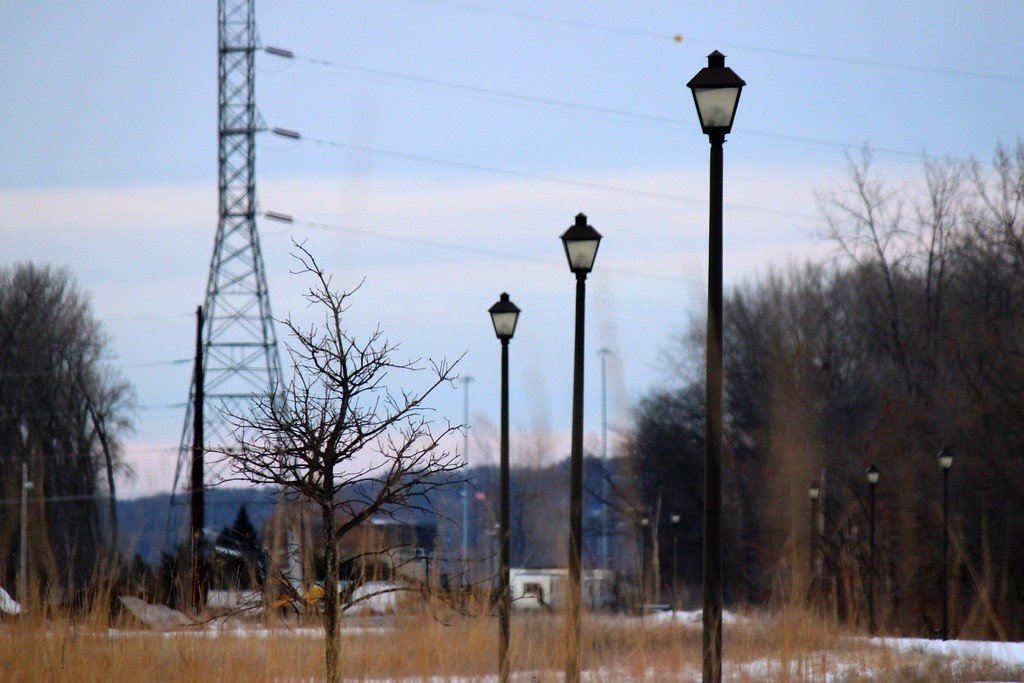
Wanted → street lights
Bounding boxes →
[487,291,522,681]
[688,50,749,683]
[478,440,970,650]
[559,213,603,683]
